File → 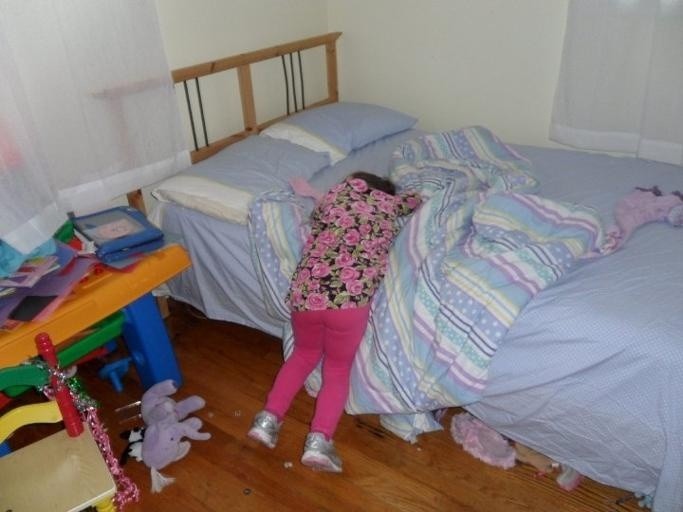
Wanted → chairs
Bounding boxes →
[0,331,116,512]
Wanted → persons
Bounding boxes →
[247,172,421,474]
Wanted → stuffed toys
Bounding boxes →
[141,378,211,493]
[120,427,144,465]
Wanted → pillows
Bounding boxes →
[150,134,330,226]
[259,102,419,167]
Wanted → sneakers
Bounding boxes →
[246,410,283,449]
[301,431,342,473]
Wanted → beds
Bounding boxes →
[90,32,683,512]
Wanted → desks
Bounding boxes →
[0,242,194,391]
